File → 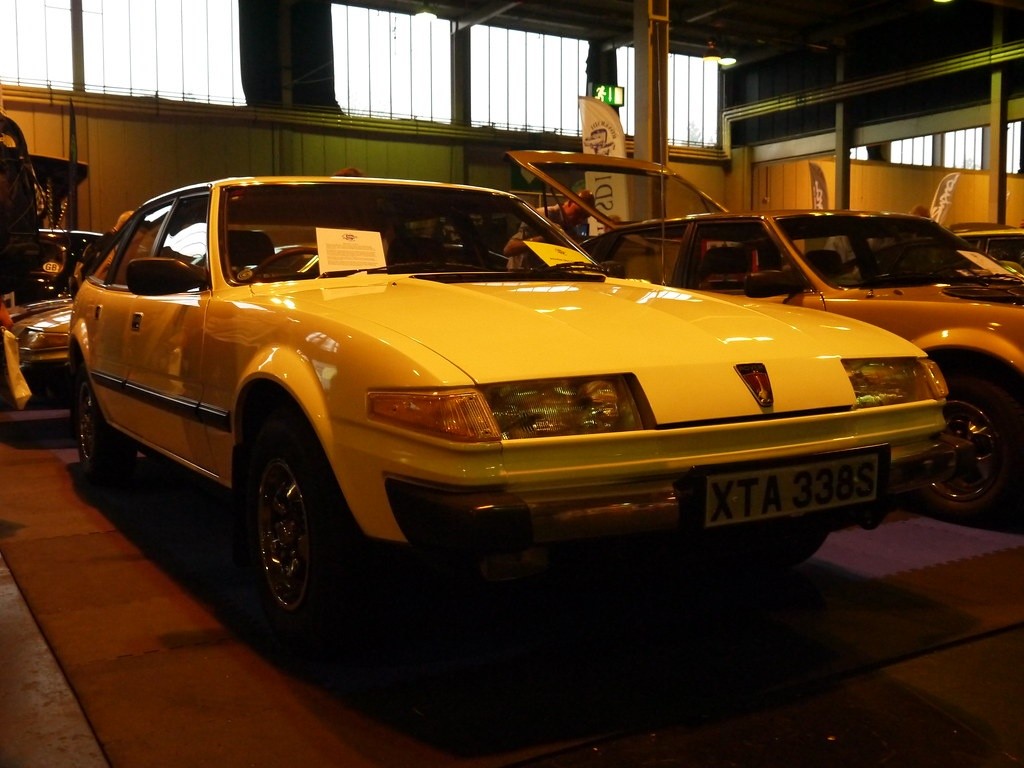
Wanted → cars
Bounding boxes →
[841,221,1024,278]
[63,174,978,646]
[502,149,1024,526]
[0,155,104,400]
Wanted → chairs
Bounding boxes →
[757,241,781,269]
[385,237,447,273]
[702,244,752,290]
[805,249,844,276]
[227,229,277,279]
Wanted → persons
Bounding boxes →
[502,190,594,272]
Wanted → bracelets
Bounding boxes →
[530,237,533,242]
